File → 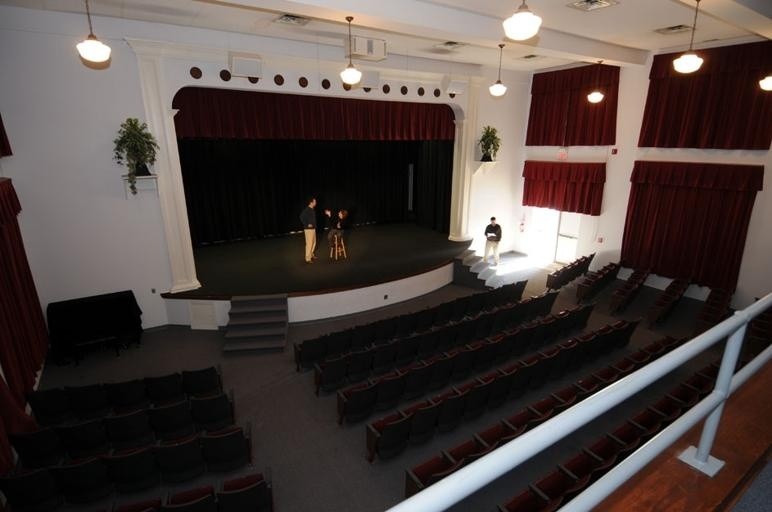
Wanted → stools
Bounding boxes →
[329,234,347,261]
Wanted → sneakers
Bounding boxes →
[312,256,319,259]
[307,261,314,264]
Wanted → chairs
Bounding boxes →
[2,362,278,509]
[546,260,771,359]
[293,279,724,510]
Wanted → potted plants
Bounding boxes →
[114,114,159,194]
[476,123,500,164]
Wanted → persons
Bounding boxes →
[484,217,502,265]
[299,196,320,264]
[324,209,348,248]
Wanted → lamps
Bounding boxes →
[669,0,706,77]
[586,60,609,106]
[339,15,363,86]
[488,44,509,97]
[499,0,545,43]
[73,0,114,71]
